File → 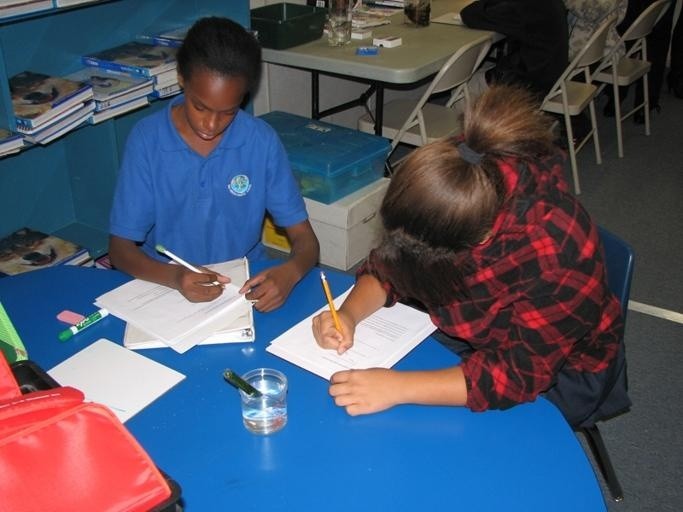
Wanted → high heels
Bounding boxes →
[666,73,683,98]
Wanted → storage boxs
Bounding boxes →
[258,111,390,206]
[262,179,400,272]
[250,2,326,51]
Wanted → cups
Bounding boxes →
[237,367,289,437]
[328,15,352,48]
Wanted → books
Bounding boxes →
[0,28,258,157]
[0,227,89,277]
[80,257,95,268]
[0,0,99,25]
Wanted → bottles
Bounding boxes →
[404,0,431,29]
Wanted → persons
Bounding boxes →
[666,5,683,100]
[108,17,320,312]
[602,0,677,123]
[563,0,630,86]
[312,83,624,433]
[460,0,569,113]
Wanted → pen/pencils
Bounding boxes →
[320,271,344,336]
[223,368,263,398]
[155,245,226,289]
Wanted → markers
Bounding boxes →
[58,307,109,342]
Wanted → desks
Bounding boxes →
[262,0,505,134]
[0,259,610,512]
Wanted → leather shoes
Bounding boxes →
[604,95,627,117]
[634,100,658,125]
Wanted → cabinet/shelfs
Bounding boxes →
[0,0,254,276]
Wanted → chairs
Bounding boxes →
[539,16,615,196]
[574,226,634,502]
[358,36,491,178]
[590,1,669,158]
